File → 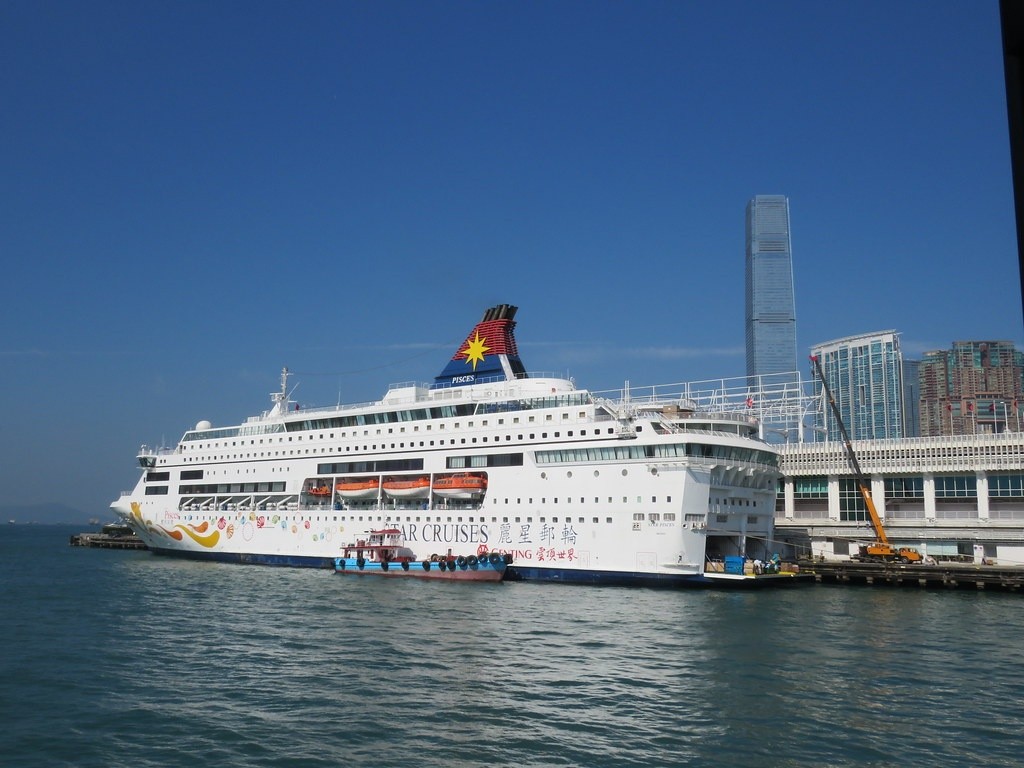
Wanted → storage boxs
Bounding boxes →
[744,569,754,575]
[717,563,724,572]
[744,563,755,569]
[781,563,792,572]
[707,562,718,571]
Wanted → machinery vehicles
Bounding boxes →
[810,355,922,563]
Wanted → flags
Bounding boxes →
[988,404,994,412]
[967,404,973,411]
[948,405,951,411]
[1014,401,1017,407]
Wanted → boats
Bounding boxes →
[334,529,506,581]
[108,304,781,589]
